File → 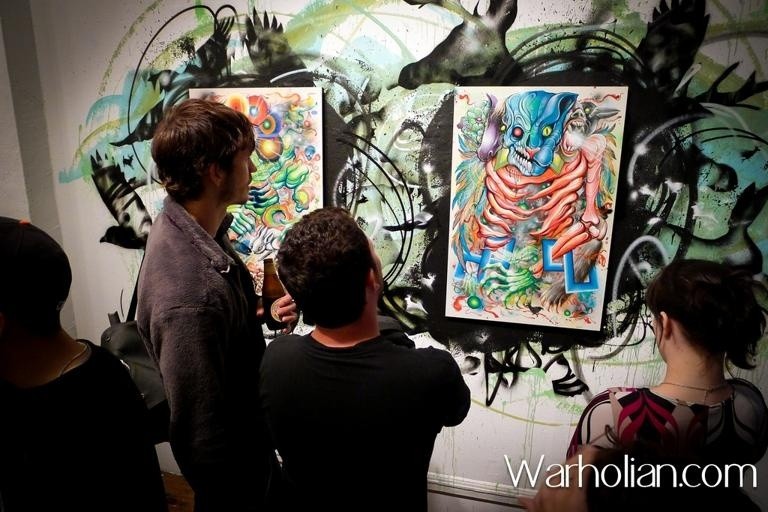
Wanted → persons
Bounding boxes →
[138,99,298,512]
[256,206,470,512]
[518,259,768,512]
[0,216,168,512]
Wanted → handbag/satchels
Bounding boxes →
[98,309,173,450]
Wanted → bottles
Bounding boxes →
[261,258,288,330]
